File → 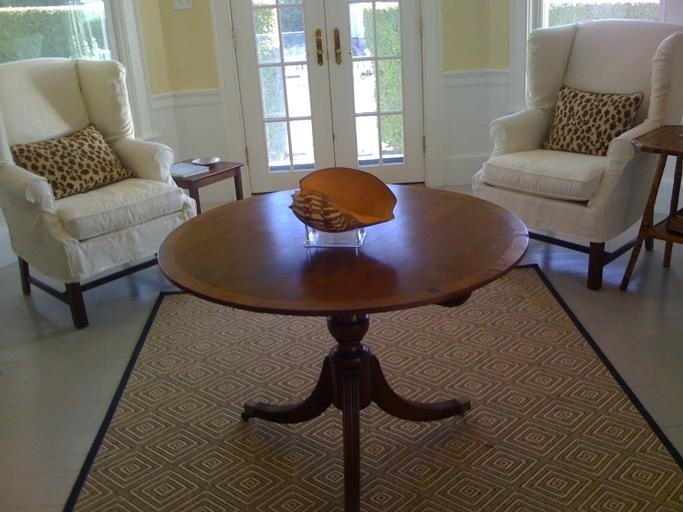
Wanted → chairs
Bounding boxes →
[468,17,682,292]
[0,59,198,329]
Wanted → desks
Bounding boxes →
[155,181,531,511]
[171,157,245,215]
[618,125,683,293]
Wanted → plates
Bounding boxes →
[192,156,221,165]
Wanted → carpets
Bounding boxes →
[64,262,682,512]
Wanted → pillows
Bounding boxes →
[541,81,645,156]
[10,122,139,203]
